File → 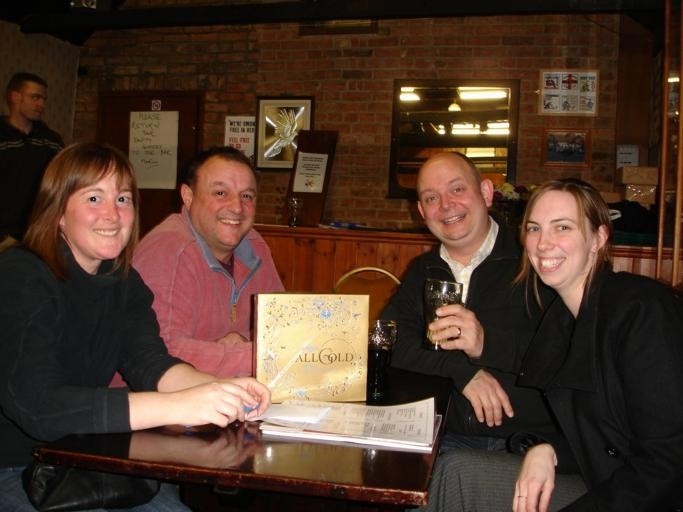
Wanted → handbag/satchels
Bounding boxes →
[28,462,161,511]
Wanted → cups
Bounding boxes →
[286,197,303,227]
[368,320,398,386]
[424,278,464,352]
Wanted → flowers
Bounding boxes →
[492,184,528,213]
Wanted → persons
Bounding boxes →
[0,73,66,253]
[0,139,271,512]
[413,178,683,512]
[108,145,286,389]
[377,151,558,456]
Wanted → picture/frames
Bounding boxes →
[541,127,592,171]
[254,95,314,173]
[537,68,599,116]
[220,112,257,170]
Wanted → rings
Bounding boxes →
[517,496,527,499]
[457,328,461,336]
